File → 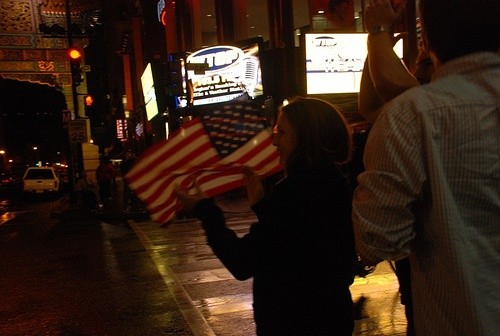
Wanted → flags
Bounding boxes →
[124,89,284,228]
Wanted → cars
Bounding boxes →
[23,166,59,200]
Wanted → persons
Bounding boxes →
[94,136,140,212]
[173,97,360,336]
[356,1,436,336]
[351,1,500,336]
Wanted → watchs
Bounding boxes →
[367,25,392,34]
